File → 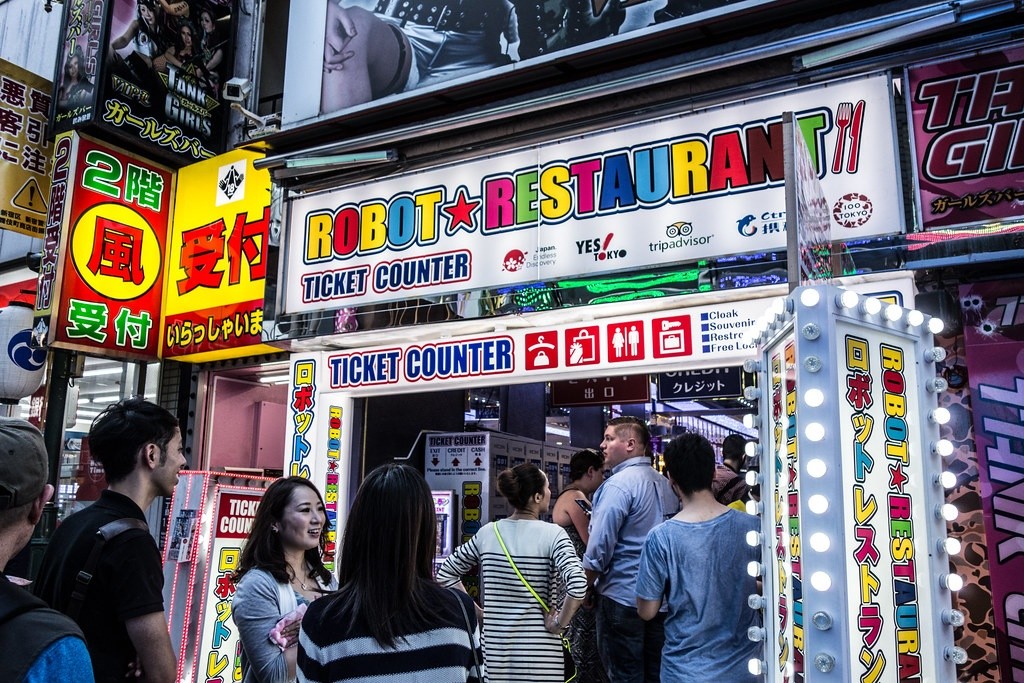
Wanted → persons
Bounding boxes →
[109,0,230,104]
[319,0,712,116]
[29,396,188,683]
[543,416,765,683]
[437,463,588,682]
[295,464,486,683]
[0,416,95,683]
[58,46,92,106]
[231,476,338,683]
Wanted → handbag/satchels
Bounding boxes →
[562,639,578,683]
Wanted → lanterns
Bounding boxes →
[0,301,46,405]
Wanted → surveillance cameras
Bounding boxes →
[222,78,252,102]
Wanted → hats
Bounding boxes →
[0,417,49,510]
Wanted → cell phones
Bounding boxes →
[574,499,592,516]
